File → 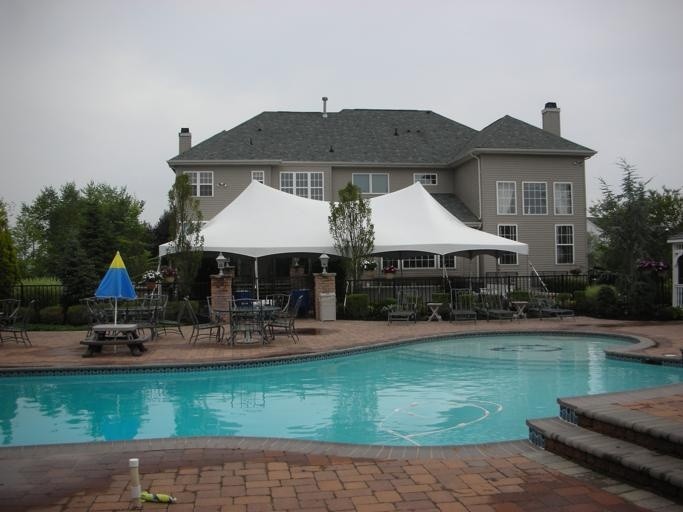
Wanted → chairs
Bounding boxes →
[476,287,515,322]
[448,288,478,326]
[387,287,419,326]
[0,299,37,349]
[528,286,577,322]
[80,292,304,358]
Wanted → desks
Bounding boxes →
[427,302,444,322]
[512,300,528,321]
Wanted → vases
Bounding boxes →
[166,276,176,284]
[145,281,156,290]
[383,272,394,280]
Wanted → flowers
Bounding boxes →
[635,259,669,272]
[162,265,178,279]
[143,270,162,283]
[383,264,397,272]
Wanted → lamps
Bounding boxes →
[215,252,227,276]
[319,254,330,274]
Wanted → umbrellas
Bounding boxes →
[96,392,142,442]
[92,250,137,352]
